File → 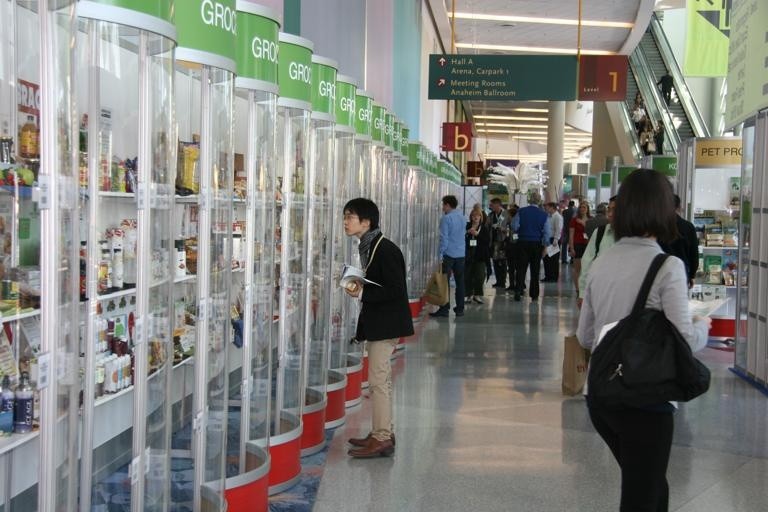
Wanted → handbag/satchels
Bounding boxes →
[424,262,451,308]
[588,255,710,401]
[562,337,585,397]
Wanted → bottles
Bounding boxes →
[1,240,136,434]
[0,114,39,164]
[174,239,187,278]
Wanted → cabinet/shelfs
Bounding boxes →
[587,101,768,393]
[1,1,462,512]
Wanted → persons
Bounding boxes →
[343,198,415,458]
[575,169,714,511]
[657,194,700,289]
[428,195,468,317]
[577,195,617,309]
[464,192,578,304]
[631,93,664,155]
[585,204,610,240]
[656,70,673,106]
[568,201,595,300]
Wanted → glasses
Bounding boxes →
[342,214,360,221]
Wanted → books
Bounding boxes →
[339,264,383,293]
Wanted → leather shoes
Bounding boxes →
[348,433,397,447]
[540,277,557,283]
[464,280,538,304]
[347,440,395,457]
[454,304,464,317]
[429,308,449,317]
